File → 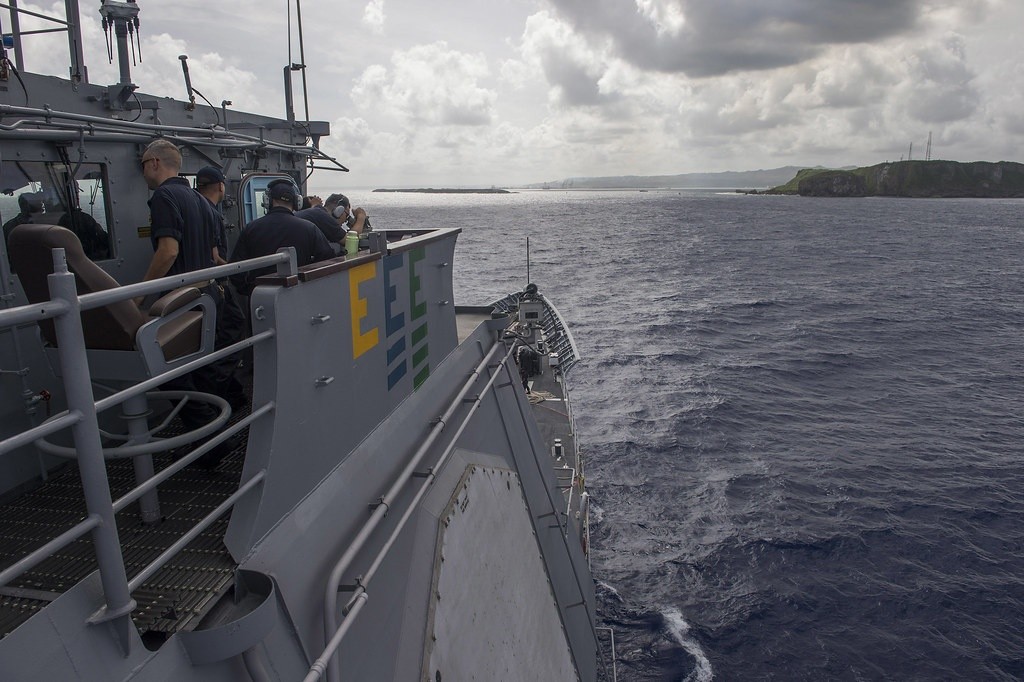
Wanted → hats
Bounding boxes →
[271,183,295,202]
[196,166,225,186]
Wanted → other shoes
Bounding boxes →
[215,353,240,366]
[173,443,220,470]
[211,376,249,417]
[216,425,242,458]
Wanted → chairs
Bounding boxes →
[7,223,232,523]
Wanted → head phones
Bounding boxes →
[261,178,303,211]
[331,195,346,219]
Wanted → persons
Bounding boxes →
[2,171,110,274]
[132,138,241,469]
[190,162,366,397]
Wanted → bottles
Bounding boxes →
[344,230,359,254]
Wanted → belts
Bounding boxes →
[172,278,215,291]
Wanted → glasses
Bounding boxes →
[141,158,160,170]
[344,211,351,220]
